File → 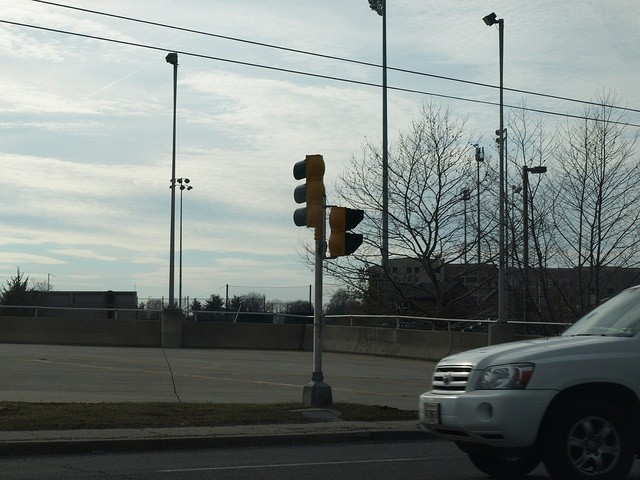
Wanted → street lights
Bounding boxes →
[511,185,522,268]
[495,128,509,266]
[165,51,178,308]
[475,146,484,264]
[368,1,388,275]
[522,165,547,333]
[168,176,193,310]
[482,12,507,324]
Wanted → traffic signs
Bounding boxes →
[462,189,469,263]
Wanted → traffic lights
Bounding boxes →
[293,154,326,228]
[330,207,363,259]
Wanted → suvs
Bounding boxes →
[418,283,639,480]
